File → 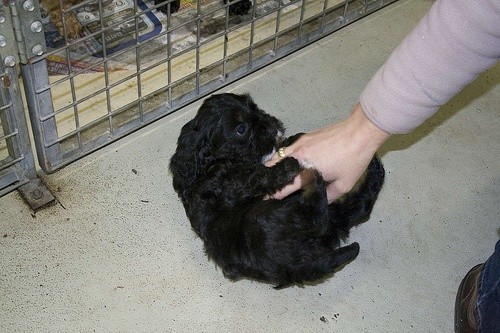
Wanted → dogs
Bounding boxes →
[170,93,385,289]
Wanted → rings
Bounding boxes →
[278,146,285,158]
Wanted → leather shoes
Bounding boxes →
[455,262,485,333]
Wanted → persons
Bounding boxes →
[257,0,500,333]
